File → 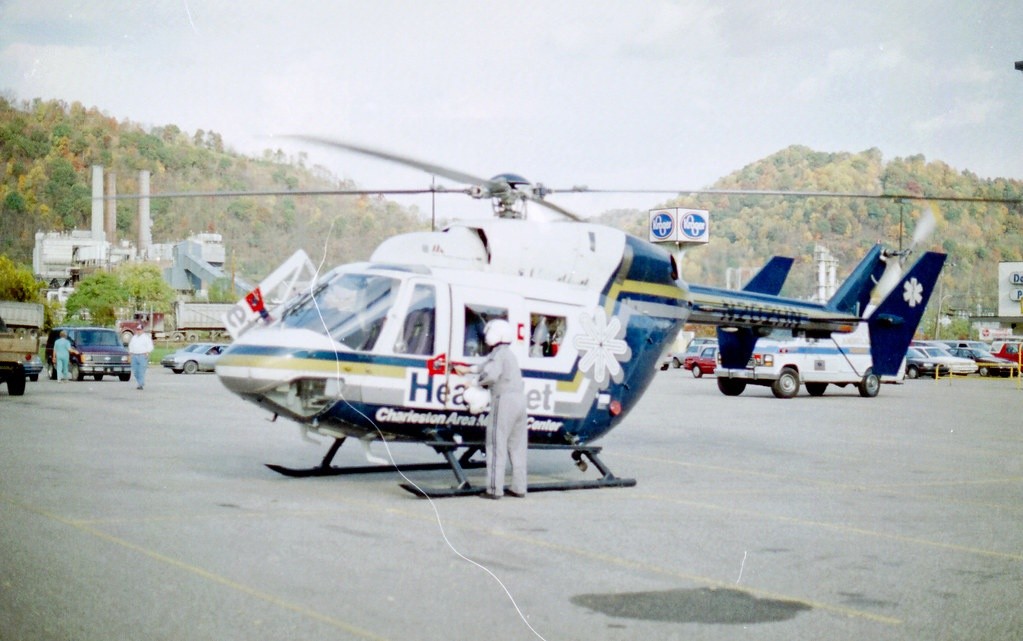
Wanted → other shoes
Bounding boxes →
[504,489,524,498]
[480,492,500,500]
[136,386,144,390]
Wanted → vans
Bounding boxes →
[716,323,879,397]
[46,324,134,382]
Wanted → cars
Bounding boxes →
[672,336,719,378]
[162,339,227,375]
[906,341,1019,386]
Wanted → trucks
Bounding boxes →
[0,298,43,396]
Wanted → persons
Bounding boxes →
[454,317,527,499]
[128,324,154,390]
[52,331,79,384]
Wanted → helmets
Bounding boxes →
[483,319,513,346]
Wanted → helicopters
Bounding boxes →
[80,132,881,500]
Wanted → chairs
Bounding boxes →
[404,310,435,355]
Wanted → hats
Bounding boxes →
[136,324,143,330]
